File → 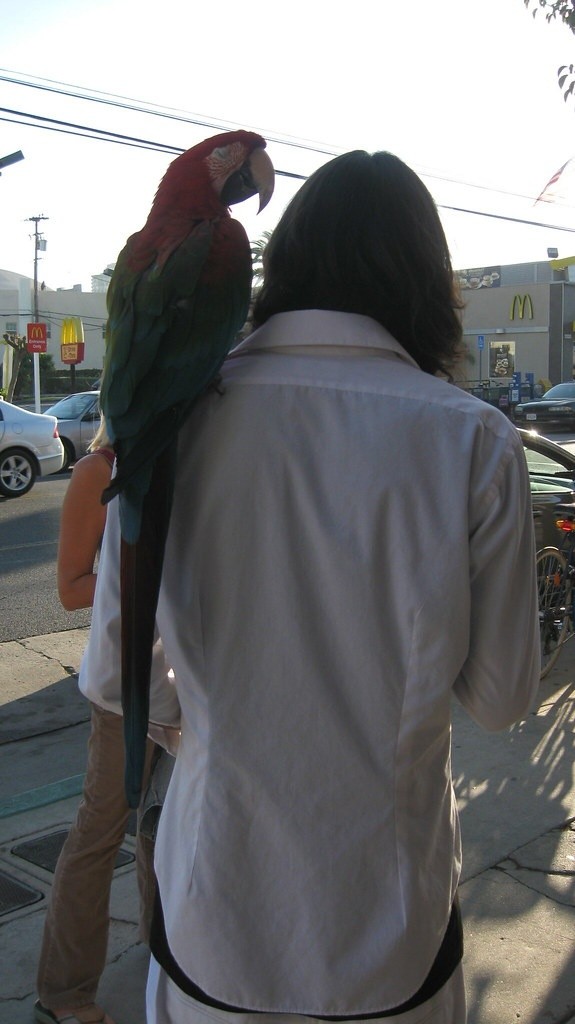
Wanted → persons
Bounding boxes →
[31,407,180,1024]
[79,151,540,1023]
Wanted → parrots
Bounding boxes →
[101,130,274,807]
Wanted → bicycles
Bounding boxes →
[519,504,575,678]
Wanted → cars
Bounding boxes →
[41,391,113,473]
[516,428,575,636]
[0,400,65,499]
[515,382,575,433]
